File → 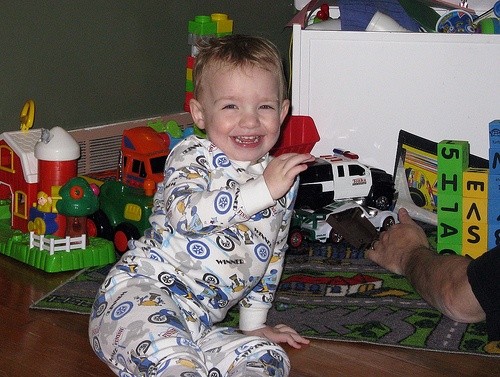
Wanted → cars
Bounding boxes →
[291,147,398,211]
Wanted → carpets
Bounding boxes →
[28,229,500,358]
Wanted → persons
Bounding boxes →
[364,208,499,324]
[88,35,316,376]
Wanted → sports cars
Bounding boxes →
[294,199,399,246]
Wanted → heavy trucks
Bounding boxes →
[87,124,173,254]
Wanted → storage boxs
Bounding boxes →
[291,23,500,174]
